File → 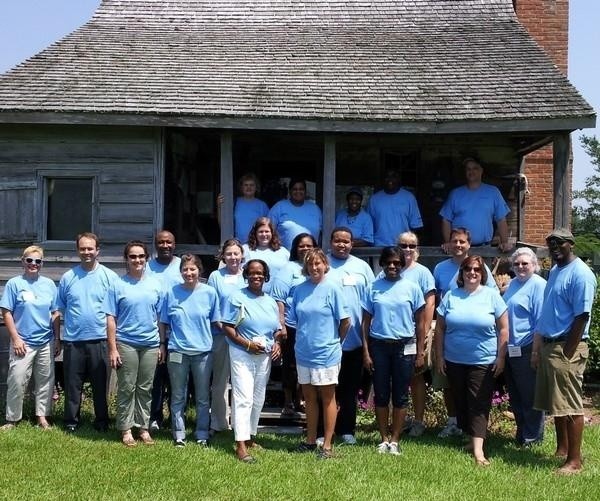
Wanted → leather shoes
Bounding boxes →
[64,423,78,433]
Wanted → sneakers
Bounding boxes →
[342,433,357,444]
[283,442,317,455]
[196,439,209,450]
[399,417,426,437]
[316,445,334,463]
[175,439,186,448]
[437,420,463,440]
[234,439,261,465]
[36,421,51,432]
[0,422,18,432]
[149,419,162,433]
[376,441,402,456]
[279,401,306,416]
[315,436,325,449]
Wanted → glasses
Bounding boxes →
[23,257,43,266]
[463,266,481,272]
[548,240,569,245]
[126,253,146,259]
[398,243,417,250]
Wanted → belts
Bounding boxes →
[542,334,588,344]
[469,240,493,248]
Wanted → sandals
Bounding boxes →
[137,430,155,446]
[119,430,138,448]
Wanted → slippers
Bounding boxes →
[474,456,491,469]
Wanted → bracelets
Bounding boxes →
[160,342,167,346]
[531,351,542,358]
[54,338,60,341]
[245,340,252,353]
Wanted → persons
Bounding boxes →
[98,238,169,450]
[205,237,249,439]
[436,155,514,256]
[264,174,325,249]
[313,225,376,449]
[159,253,221,451]
[280,247,353,462]
[357,246,428,460]
[374,231,439,439]
[269,232,320,419]
[502,245,548,452]
[528,225,598,479]
[432,253,511,470]
[57,231,121,435]
[362,162,425,246]
[0,244,62,434]
[432,226,502,441]
[222,258,283,467]
[332,186,375,249]
[147,229,187,434]
[214,173,270,245]
[240,216,291,297]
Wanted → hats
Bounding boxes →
[545,227,576,243]
[346,187,363,198]
